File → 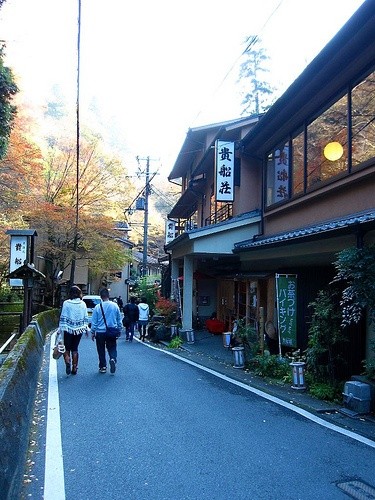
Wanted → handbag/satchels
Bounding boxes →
[106,327,120,337]
[52,333,65,359]
[122,315,130,327]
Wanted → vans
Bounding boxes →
[82,296,103,329]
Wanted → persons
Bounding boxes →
[138,297,149,341]
[55,286,89,375]
[154,284,160,297]
[113,296,122,312]
[123,297,140,342]
[90,289,123,373]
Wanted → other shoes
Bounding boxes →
[109,359,115,374]
[99,367,106,373]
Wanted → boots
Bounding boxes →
[71,351,79,375]
[63,351,71,375]
